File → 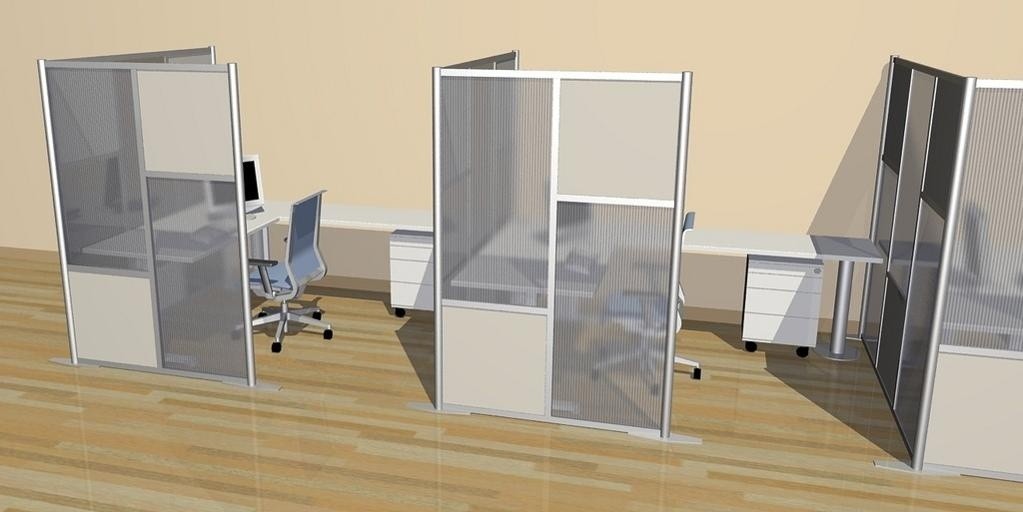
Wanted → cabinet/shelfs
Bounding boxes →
[386,230,433,317]
[740,254,823,358]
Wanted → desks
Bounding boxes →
[80,198,434,342]
[447,212,887,363]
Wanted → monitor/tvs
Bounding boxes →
[204,154,265,214]
[965,205,990,276]
[544,178,594,244]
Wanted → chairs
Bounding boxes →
[230,188,333,352]
[586,209,702,395]
